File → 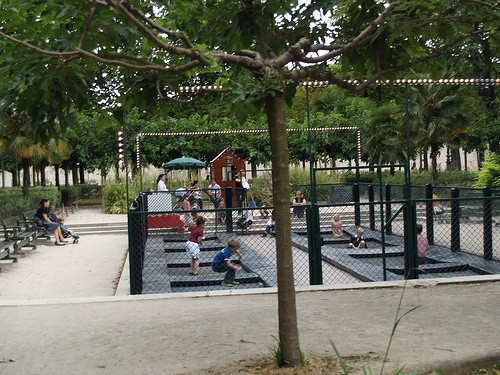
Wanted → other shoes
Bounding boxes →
[229,281,239,285]
[189,272,198,276]
[60,240,69,242]
[73,235,79,239]
[55,242,66,245]
[221,281,233,287]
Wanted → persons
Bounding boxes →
[156,162,446,286]
[58,218,79,238]
[35,198,68,246]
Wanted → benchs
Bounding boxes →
[0,216,51,273]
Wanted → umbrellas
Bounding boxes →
[163,156,206,188]
